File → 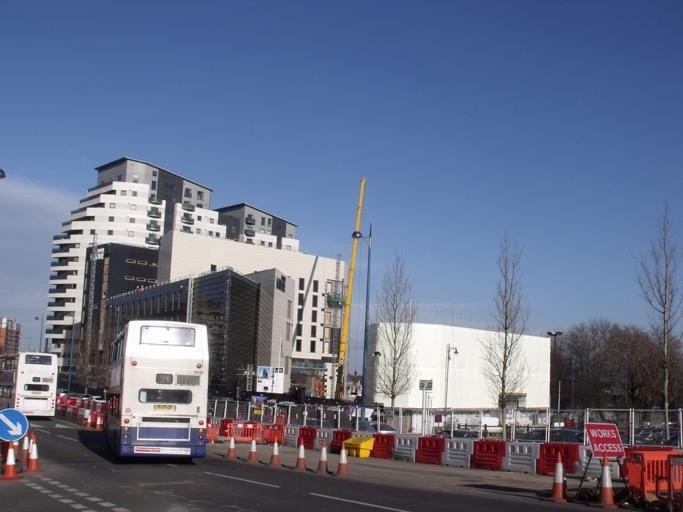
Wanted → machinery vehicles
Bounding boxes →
[264,171,386,422]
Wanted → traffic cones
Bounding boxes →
[245,438,259,463]
[335,441,352,478]
[290,435,312,472]
[0,428,39,481]
[266,436,282,467]
[82,414,94,428]
[313,438,333,475]
[94,412,102,432]
[582,450,619,509]
[546,450,565,504]
[223,436,242,461]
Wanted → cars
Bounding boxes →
[638,423,678,446]
[57,390,105,423]
[363,424,398,436]
[518,429,583,443]
[434,429,481,438]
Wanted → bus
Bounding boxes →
[0,350,60,420]
[102,317,213,466]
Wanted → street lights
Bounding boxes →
[105,304,120,332]
[63,311,74,393]
[442,343,458,429]
[361,350,383,406]
[348,223,373,424]
[569,362,579,410]
[545,329,563,358]
[0,167,7,181]
[34,314,43,352]
